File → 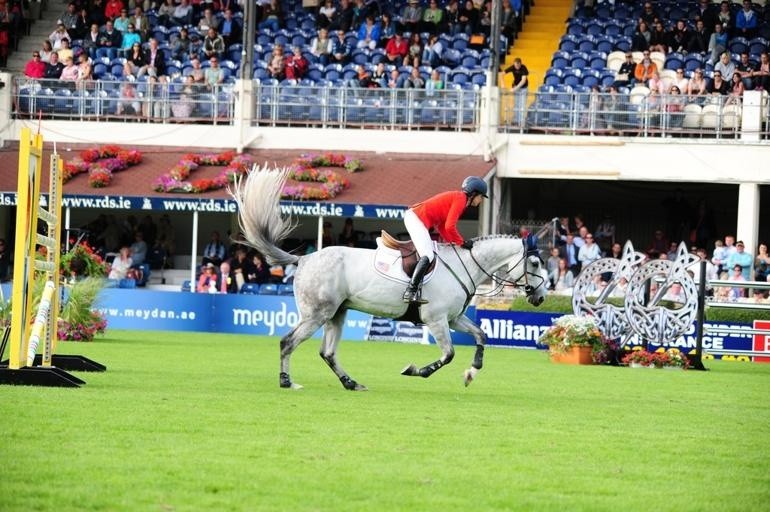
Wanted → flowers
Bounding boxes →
[620,346,655,366]
[649,347,690,369]
[539,309,613,366]
[50,239,110,342]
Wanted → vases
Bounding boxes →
[550,344,594,366]
[663,362,685,370]
[630,359,654,369]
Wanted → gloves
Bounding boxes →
[461,240,473,250]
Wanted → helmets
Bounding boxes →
[462,176,488,198]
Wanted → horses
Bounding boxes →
[226,161,549,391]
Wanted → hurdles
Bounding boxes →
[0,127,107,387]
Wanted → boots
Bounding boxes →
[403,256,428,304]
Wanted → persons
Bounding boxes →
[22,1,242,124]
[43,217,179,288]
[641,227,770,302]
[401,175,488,304]
[522,217,620,298]
[580,0,770,135]
[268,1,530,125]
[196,226,318,292]
